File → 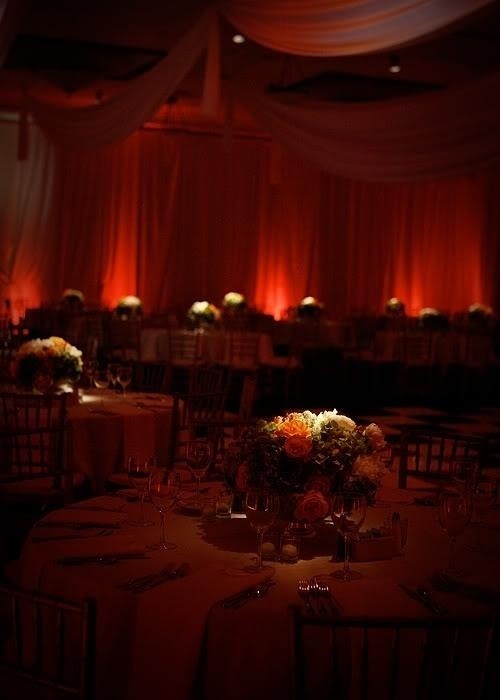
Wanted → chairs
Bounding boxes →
[0,291,498,700]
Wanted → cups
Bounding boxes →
[216,486,233,518]
[168,468,190,509]
[278,534,300,566]
[383,514,408,547]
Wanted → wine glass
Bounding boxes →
[185,439,212,511]
[369,444,396,508]
[215,437,240,497]
[434,454,497,551]
[327,493,369,579]
[126,453,157,527]
[78,357,132,414]
[243,487,280,574]
[147,469,180,551]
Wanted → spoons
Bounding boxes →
[296,573,339,620]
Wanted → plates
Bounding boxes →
[125,388,173,409]
